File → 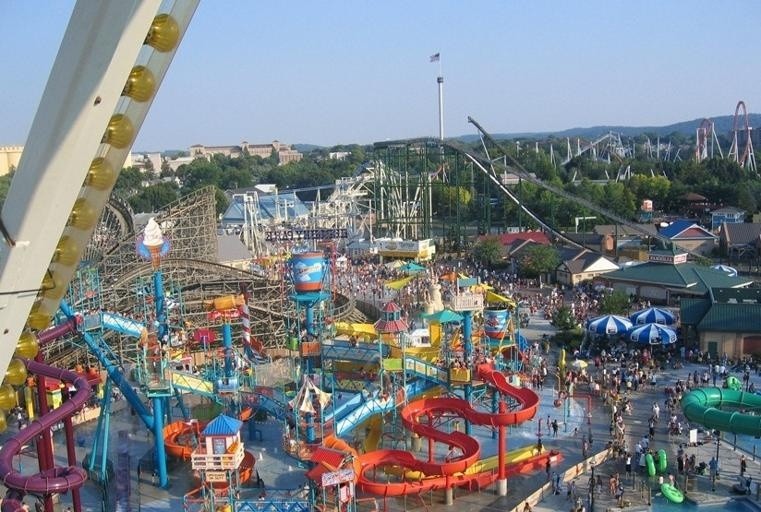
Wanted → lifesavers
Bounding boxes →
[733,484,747,495]
[646,450,667,478]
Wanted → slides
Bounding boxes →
[303,320,563,498]
[0,315,91,509]
[161,407,255,497]
[681,388,761,439]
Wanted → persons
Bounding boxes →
[22,501,30,511]
[336,252,653,403]
[527,331,761,511]
[63,506,72,512]
[17,410,23,429]
[256,488,267,509]
[151,471,161,486]
[236,490,240,499]
[88,305,146,324]
[284,481,311,500]
[446,446,457,458]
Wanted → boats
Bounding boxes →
[657,449,667,473]
[646,454,656,476]
